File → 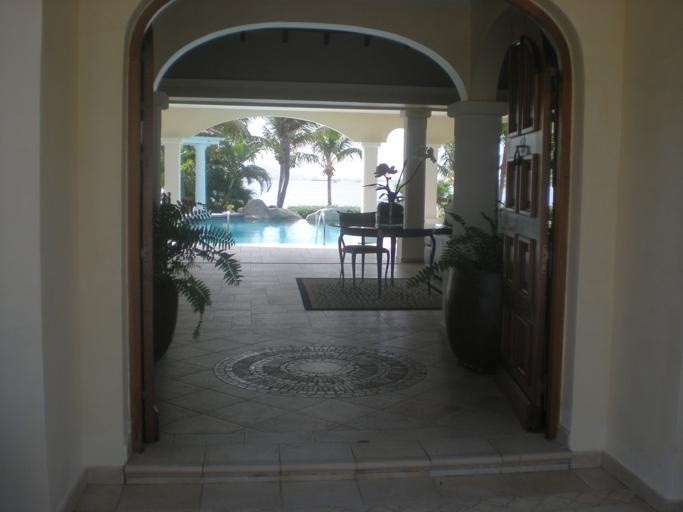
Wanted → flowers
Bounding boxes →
[363,144,436,222]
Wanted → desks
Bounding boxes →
[338,222,453,299]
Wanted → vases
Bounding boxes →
[376,201,404,228]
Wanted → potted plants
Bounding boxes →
[404,206,504,369]
[153,191,244,361]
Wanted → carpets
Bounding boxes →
[295,278,443,312]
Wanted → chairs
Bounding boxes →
[337,211,389,287]
